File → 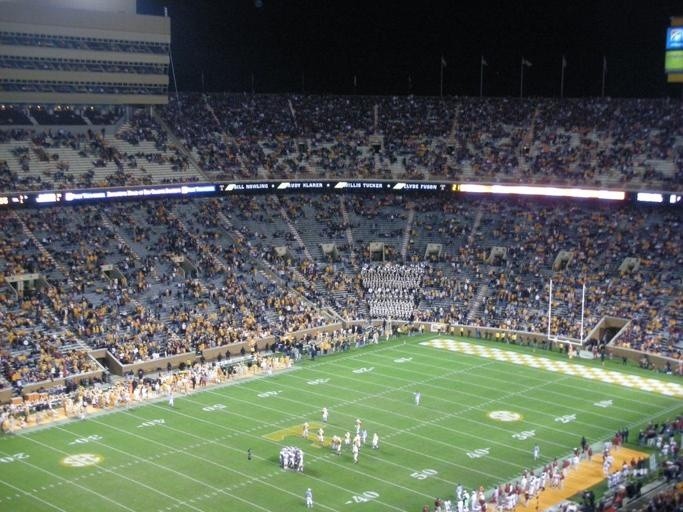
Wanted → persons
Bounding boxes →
[1,90,683,512]
